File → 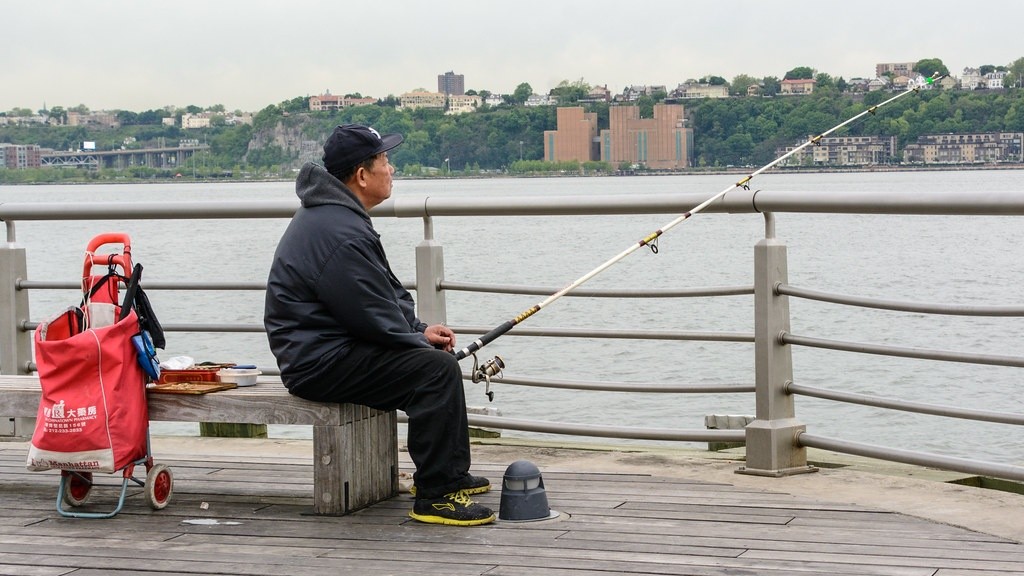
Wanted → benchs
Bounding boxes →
[0,374,398,518]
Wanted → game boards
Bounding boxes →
[146,380,238,395]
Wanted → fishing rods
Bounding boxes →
[445,71,949,403]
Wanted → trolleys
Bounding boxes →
[28,232,174,519]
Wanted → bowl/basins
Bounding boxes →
[398,473,414,492]
[216,368,263,387]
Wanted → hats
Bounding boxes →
[321,124,403,174]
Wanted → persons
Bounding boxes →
[264,123,497,527]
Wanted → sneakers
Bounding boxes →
[409,472,492,497]
[408,489,496,526]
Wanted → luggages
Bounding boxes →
[26,232,173,518]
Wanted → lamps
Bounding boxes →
[498,461,550,520]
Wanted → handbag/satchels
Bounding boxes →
[132,283,165,380]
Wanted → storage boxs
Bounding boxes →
[154,363,221,383]
